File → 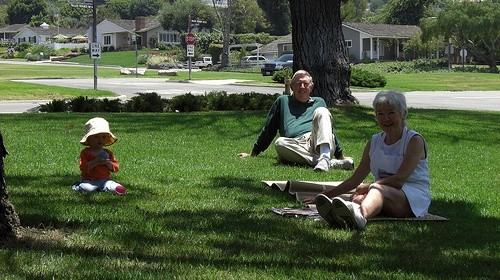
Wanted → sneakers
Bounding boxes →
[315,194,367,230]
[313,157,330,172]
[330,159,354,170]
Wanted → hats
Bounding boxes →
[81,117,117,146]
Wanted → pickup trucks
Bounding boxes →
[195,57,212,69]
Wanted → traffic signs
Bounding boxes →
[91,42,101,59]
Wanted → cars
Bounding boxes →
[244,56,265,64]
[261,54,294,76]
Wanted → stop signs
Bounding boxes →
[185,33,196,45]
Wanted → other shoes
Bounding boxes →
[115,186,126,194]
[72,182,80,190]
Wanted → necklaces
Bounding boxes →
[387,135,398,142]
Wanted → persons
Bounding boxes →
[236,69,354,172]
[302,90,433,231]
[71,117,123,194]
